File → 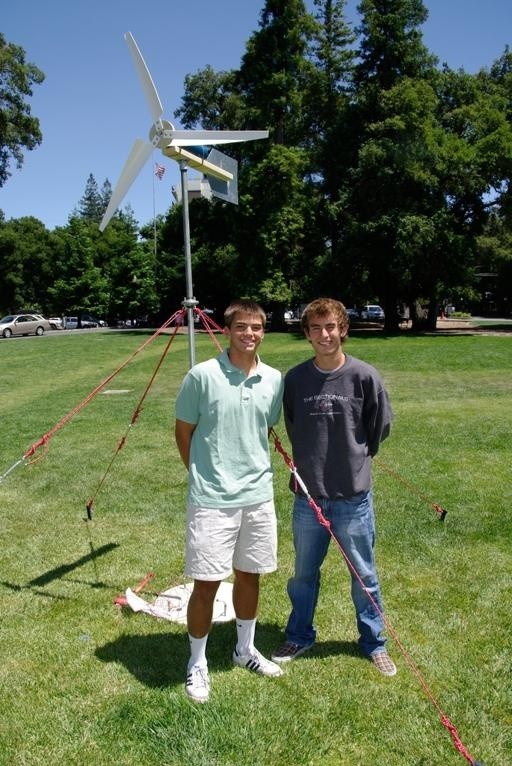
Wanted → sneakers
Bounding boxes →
[186,657,210,702]
[371,651,396,676]
[232,644,283,678]
[271,639,315,662]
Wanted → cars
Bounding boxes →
[267,308,295,320]
[361,304,386,321]
[346,308,360,320]
[47,316,109,329]
[0,313,50,338]
[116,319,139,328]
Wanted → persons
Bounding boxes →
[176,301,287,705]
[270,297,397,677]
